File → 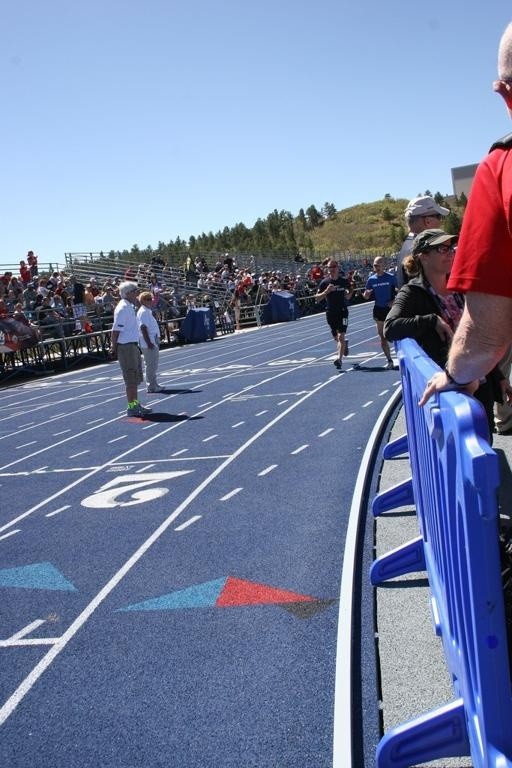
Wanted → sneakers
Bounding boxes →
[333,358,342,369]
[387,360,393,370]
[147,384,165,393]
[344,339,348,356]
[127,404,152,417]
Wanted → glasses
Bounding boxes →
[421,213,442,221]
[421,244,455,254]
[131,288,140,294]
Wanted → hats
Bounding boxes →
[373,256,384,267]
[411,229,459,260]
[404,195,450,216]
